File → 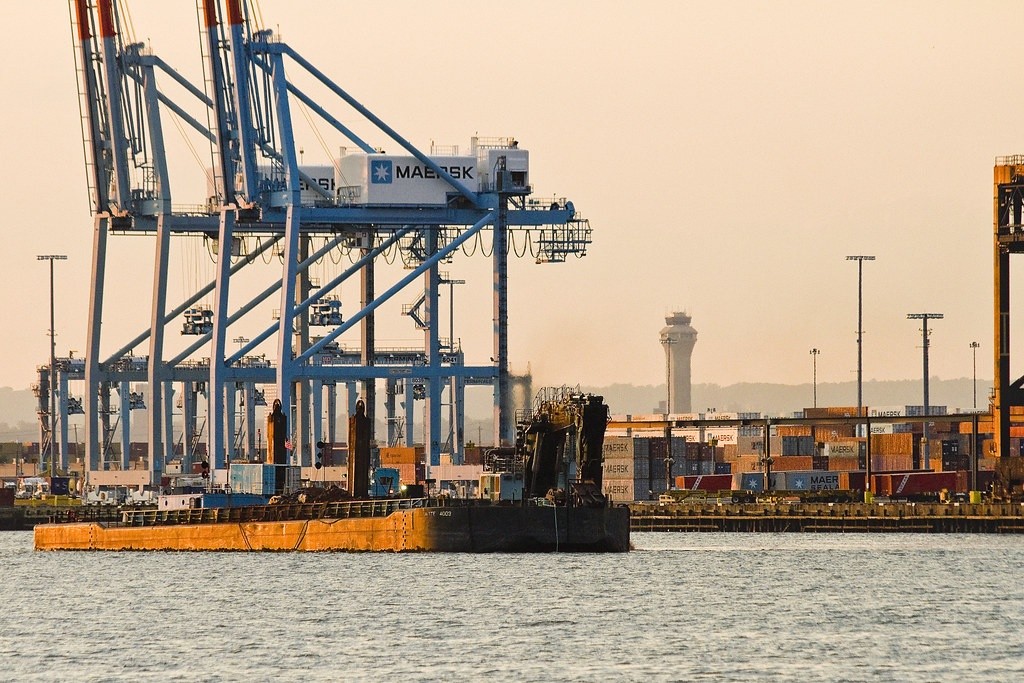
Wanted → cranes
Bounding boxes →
[25,1,604,522]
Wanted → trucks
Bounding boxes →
[5,478,50,500]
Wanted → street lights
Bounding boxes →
[660,334,684,492]
[441,278,467,453]
[37,253,70,476]
[905,313,945,470]
[845,255,877,436]
[809,348,821,408]
[970,341,982,410]
